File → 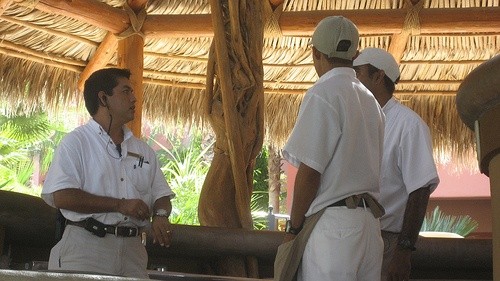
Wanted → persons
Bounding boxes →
[40,67,176,279]
[353,47,441,281]
[280,16,385,281]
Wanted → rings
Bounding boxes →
[166,230,171,233]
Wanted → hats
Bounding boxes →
[308,15,361,61]
[350,47,401,84]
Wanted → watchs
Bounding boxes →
[284,219,303,235]
[152,208,170,217]
[397,235,418,254]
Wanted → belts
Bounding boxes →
[64,219,147,237]
[327,198,370,207]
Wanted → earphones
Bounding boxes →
[102,96,106,102]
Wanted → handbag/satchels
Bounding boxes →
[273,193,385,281]
